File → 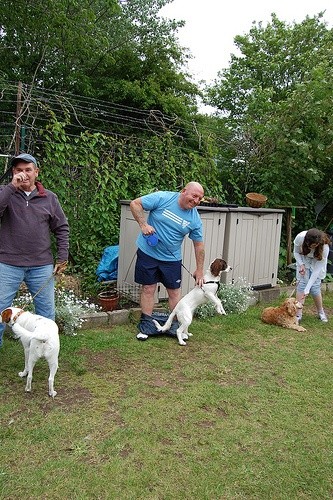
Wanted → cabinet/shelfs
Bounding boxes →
[221,212,283,289]
[117,205,227,304]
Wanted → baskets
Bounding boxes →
[246,193,268,208]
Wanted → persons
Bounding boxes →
[0,154,70,347]
[130,181,205,341]
[293,229,331,323]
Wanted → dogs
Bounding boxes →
[0,307,61,397]
[153,257,233,346]
[261,297,307,332]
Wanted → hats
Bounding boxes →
[11,154,37,168]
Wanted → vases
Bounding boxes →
[97,291,119,311]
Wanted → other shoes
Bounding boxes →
[137,332,148,340]
[296,316,302,324]
[318,312,328,322]
[180,332,189,341]
